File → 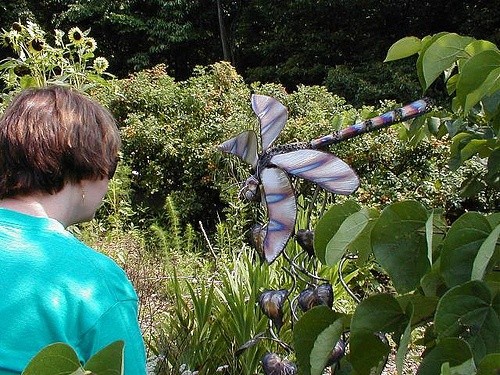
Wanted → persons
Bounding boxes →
[0,86,148,375]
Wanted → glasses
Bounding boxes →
[108,154,121,179]
